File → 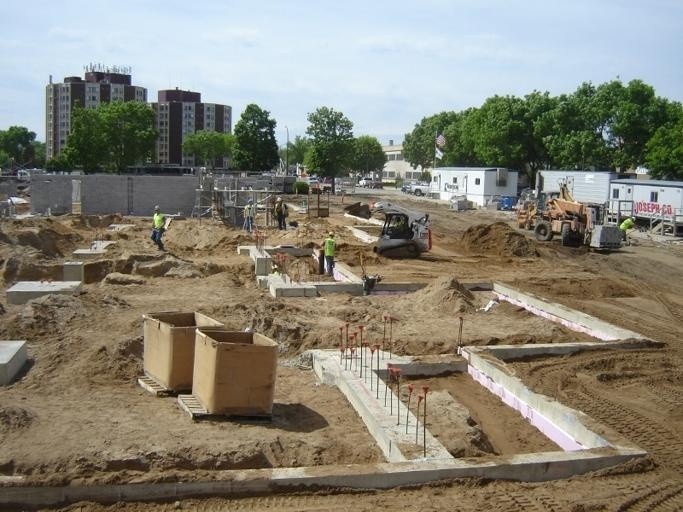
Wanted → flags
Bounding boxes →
[435,147,443,161]
[435,132,448,145]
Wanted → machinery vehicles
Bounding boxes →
[343,199,432,258]
[513,172,622,251]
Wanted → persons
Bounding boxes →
[319,231,336,274]
[618,216,641,241]
[243,199,256,234]
[272,197,289,231]
[150,204,170,250]
[392,217,404,230]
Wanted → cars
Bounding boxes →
[297,172,429,196]
